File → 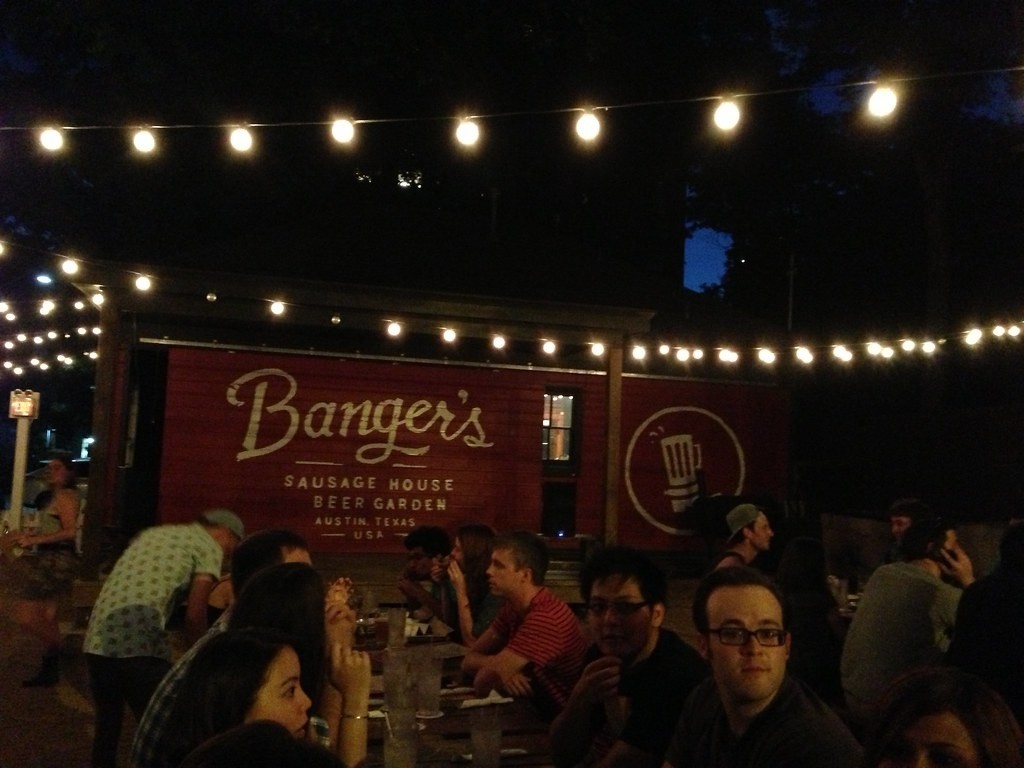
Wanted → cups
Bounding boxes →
[382,606,441,768]
[470,704,504,768]
[828,576,848,611]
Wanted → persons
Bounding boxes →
[83,484,1024,768]
[11,453,86,685]
[664,568,866,767]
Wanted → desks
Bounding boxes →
[352,621,552,768]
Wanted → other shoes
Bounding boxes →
[22,668,58,685]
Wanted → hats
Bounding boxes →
[203,508,245,540]
[727,504,761,540]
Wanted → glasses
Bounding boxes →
[589,601,649,615]
[708,627,787,646]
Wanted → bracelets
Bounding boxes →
[342,714,367,720]
